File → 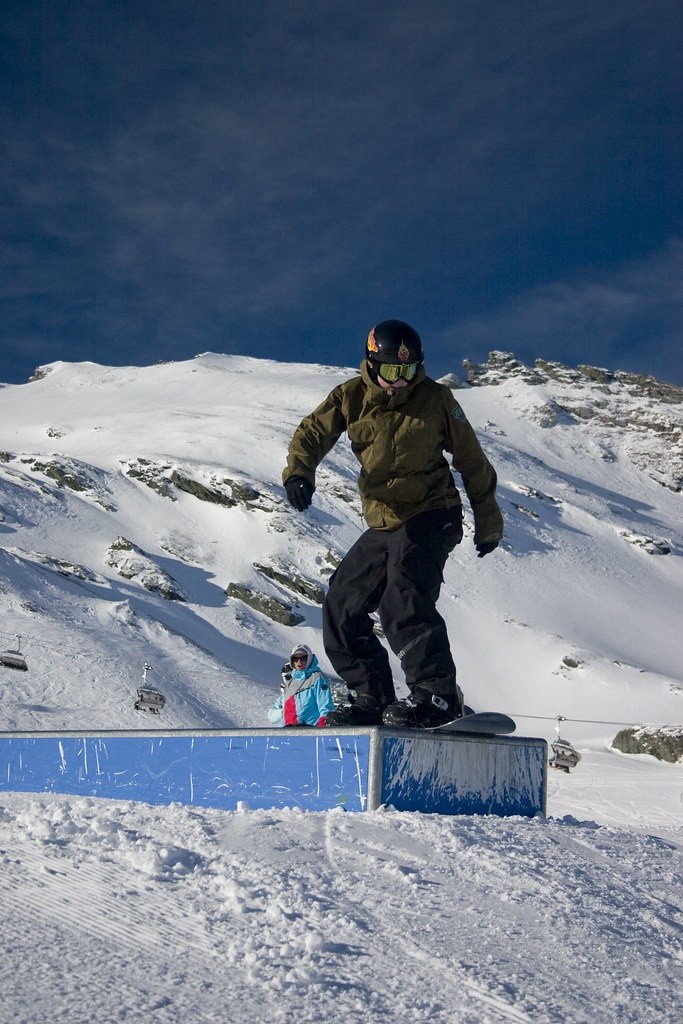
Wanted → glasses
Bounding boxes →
[292,656,308,662]
[375,361,420,384]
[282,665,290,672]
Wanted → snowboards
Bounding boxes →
[407,712,517,736]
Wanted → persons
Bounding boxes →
[267,644,335,728]
[282,320,503,728]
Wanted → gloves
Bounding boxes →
[475,541,498,558]
[285,477,314,513]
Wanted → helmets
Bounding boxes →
[365,320,423,365]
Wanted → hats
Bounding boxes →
[294,649,307,657]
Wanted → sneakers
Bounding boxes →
[326,696,390,726]
[381,689,460,728]
